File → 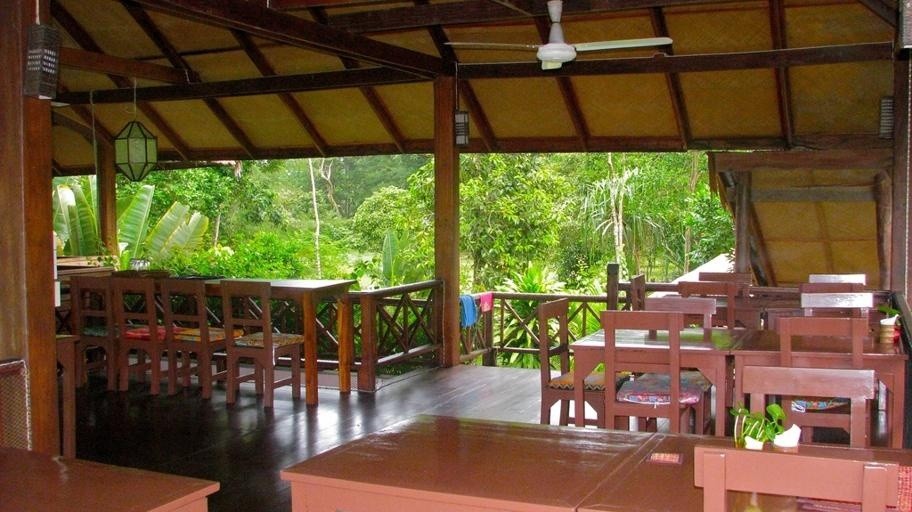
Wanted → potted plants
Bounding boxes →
[730,404,785,452]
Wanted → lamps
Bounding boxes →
[22,25,63,102]
[454,107,472,149]
[875,93,897,143]
[113,74,157,180]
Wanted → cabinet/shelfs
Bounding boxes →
[0,260,59,456]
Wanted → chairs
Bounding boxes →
[801,283,872,334]
[69,277,145,392]
[744,365,876,445]
[160,279,241,400]
[598,309,685,434]
[538,298,631,431]
[110,278,190,396]
[218,280,305,410]
[627,256,905,321]
[693,446,902,512]
[778,315,874,446]
[639,298,717,328]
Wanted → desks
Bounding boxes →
[575,427,912,511]
[574,328,910,449]
[155,278,357,405]
[2,445,221,512]
[281,414,652,511]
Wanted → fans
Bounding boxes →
[445,1,675,75]
